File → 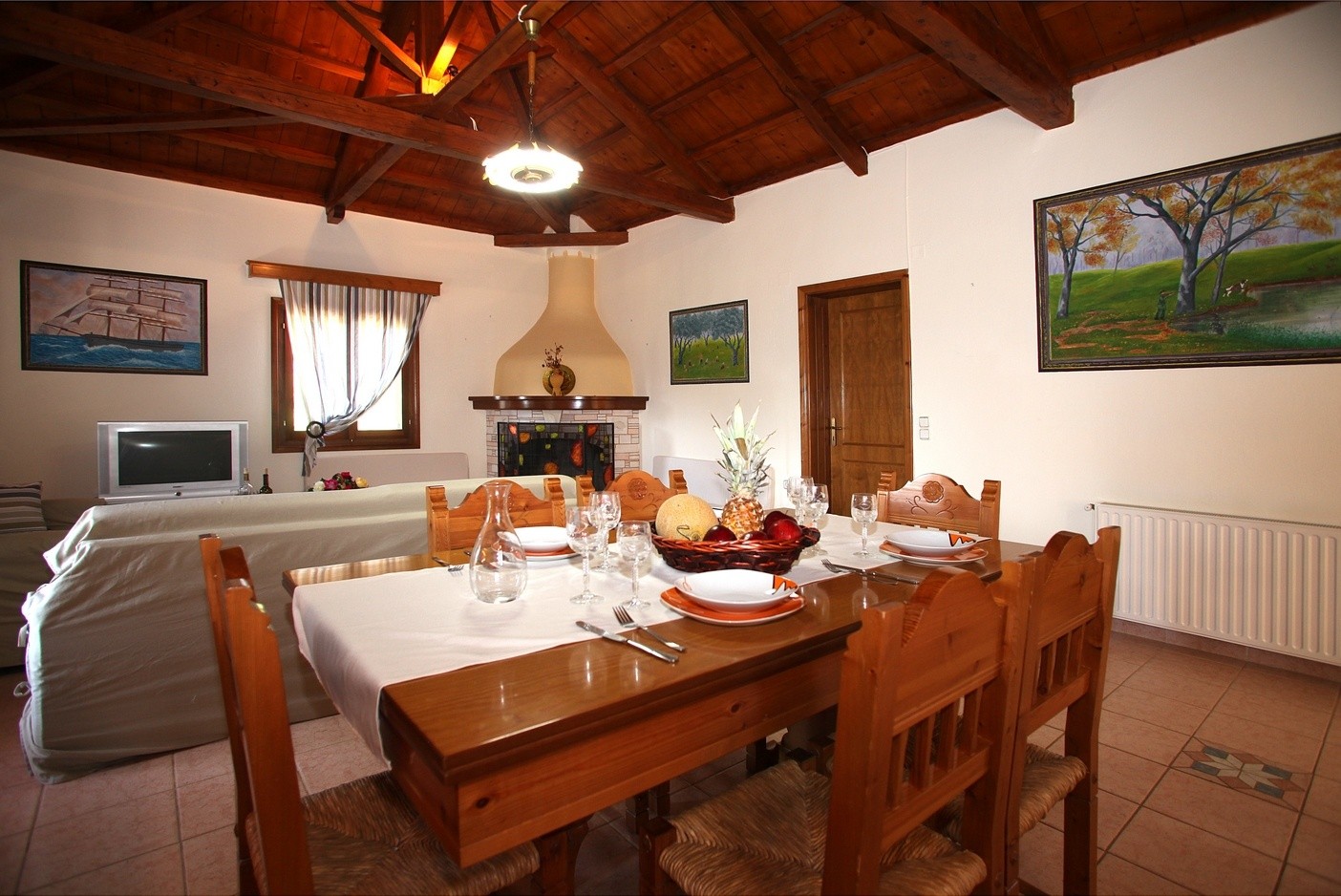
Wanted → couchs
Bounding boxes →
[13,475,578,786]
[0,499,107,668]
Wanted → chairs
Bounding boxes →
[199,468,1121,896]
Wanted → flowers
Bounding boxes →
[309,471,368,492]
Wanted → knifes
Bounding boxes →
[822,564,921,585]
[575,621,679,664]
[464,550,497,570]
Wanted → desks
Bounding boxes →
[281,508,1045,896]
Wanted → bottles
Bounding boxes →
[239,467,254,495]
[468,481,528,604]
[260,467,272,494]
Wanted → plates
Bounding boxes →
[672,569,799,614]
[496,527,573,552]
[542,363,575,394]
[879,542,989,567]
[490,543,580,561]
[882,530,978,557]
[659,587,809,627]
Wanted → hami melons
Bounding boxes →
[655,493,719,541]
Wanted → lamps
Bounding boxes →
[481,5,583,194]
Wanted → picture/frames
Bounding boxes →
[1033,133,1341,372]
[20,259,208,375]
[669,299,750,385]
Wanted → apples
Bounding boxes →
[702,510,802,543]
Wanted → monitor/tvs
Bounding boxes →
[97,422,248,505]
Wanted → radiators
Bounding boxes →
[1084,503,1341,666]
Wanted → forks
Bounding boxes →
[820,559,898,585]
[611,605,687,652]
[432,555,464,572]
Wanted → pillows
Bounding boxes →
[0,481,47,533]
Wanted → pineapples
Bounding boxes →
[711,398,774,538]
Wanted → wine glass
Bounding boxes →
[587,491,621,573]
[851,493,877,558]
[616,520,652,609]
[851,568,878,617]
[800,484,829,556]
[786,476,815,526]
[565,506,605,606]
